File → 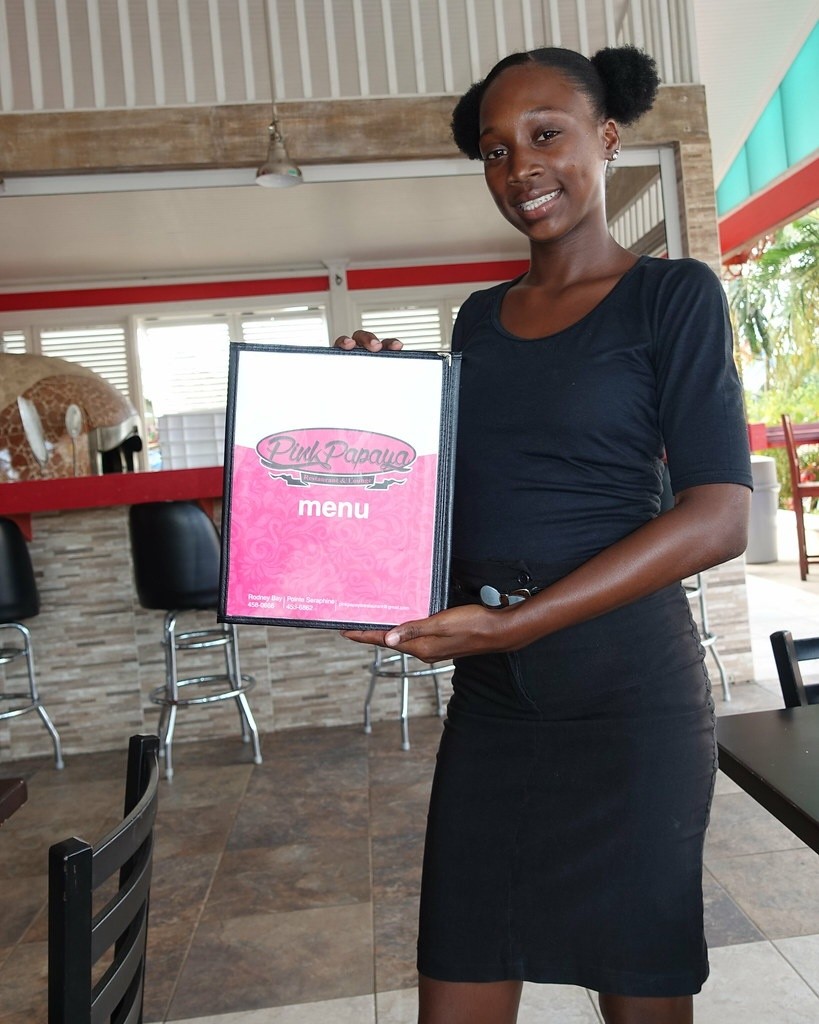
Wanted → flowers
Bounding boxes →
[797,443,819,482]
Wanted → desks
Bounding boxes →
[716,703,819,855]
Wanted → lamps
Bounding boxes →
[253,105,304,189]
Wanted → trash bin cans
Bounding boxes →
[745,454,782,565]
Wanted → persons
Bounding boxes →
[334,46,755,1024]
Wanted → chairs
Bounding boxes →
[782,414,819,581]
[47,733,160,1024]
[660,468,731,701]
[128,501,262,779]
[361,645,456,750]
[770,630,819,707]
[0,515,65,770]
